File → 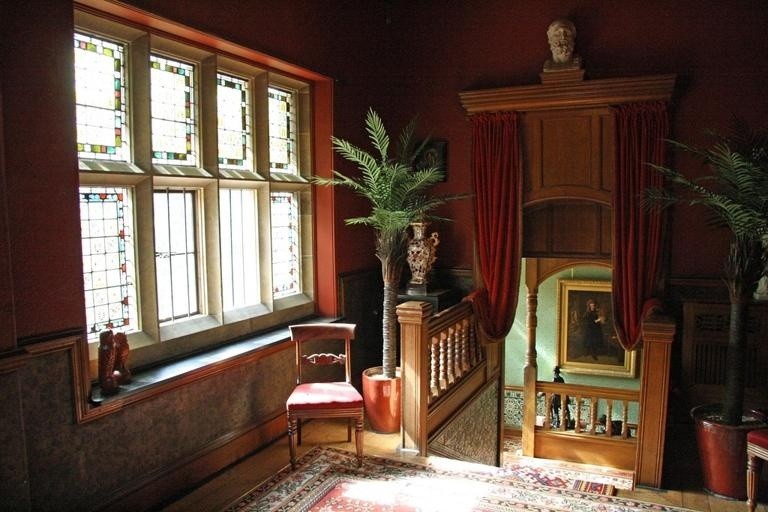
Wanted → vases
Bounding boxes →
[405,220,442,285]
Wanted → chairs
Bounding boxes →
[281,322,365,471]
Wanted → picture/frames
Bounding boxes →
[551,278,642,380]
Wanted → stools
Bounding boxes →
[742,426,767,512]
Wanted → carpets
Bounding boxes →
[220,442,696,512]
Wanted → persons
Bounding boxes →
[542,18,581,72]
[553,366,571,428]
[583,299,602,360]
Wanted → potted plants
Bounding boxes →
[630,121,767,501]
[308,104,479,436]
